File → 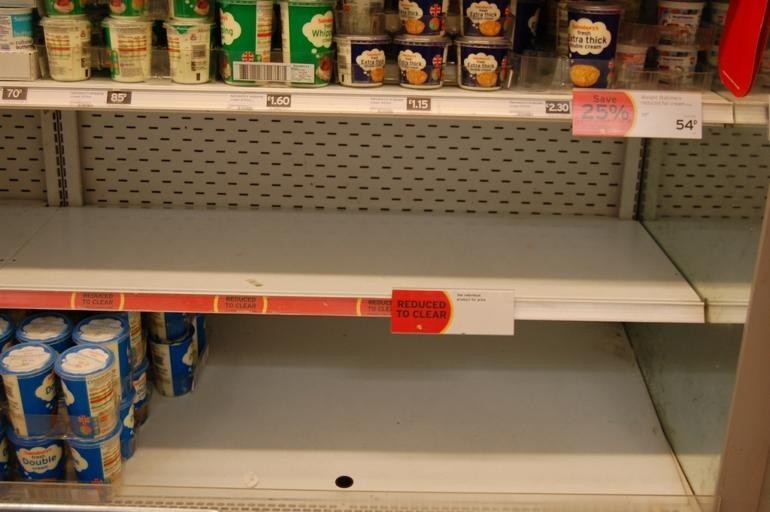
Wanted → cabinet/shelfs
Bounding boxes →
[0,78,762,512]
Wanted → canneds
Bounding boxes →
[334,0,543,89]
[39,0,334,88]
[555,2,729,86]
[0,6,33,48]
